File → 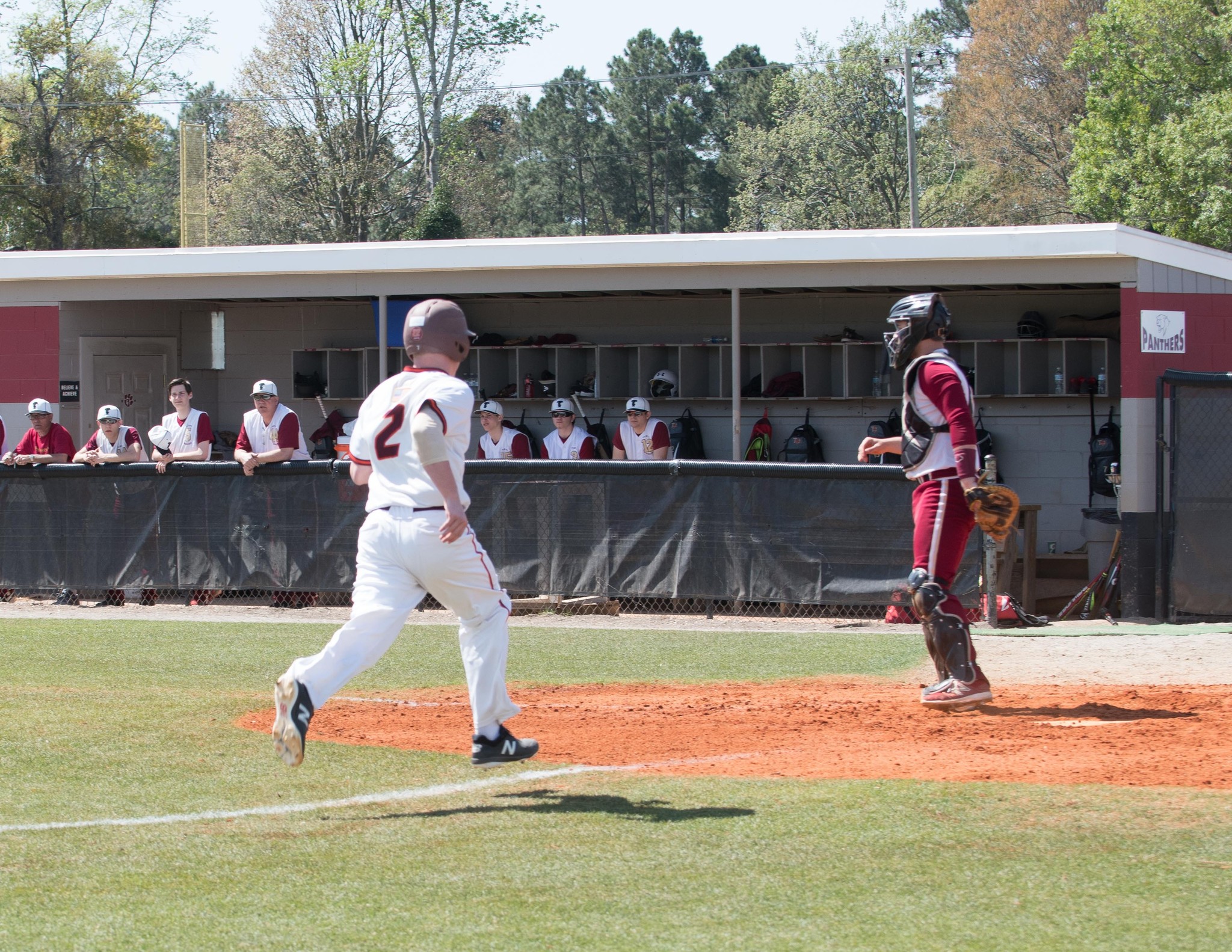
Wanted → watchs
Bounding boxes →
[30,454,34,462]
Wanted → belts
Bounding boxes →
[380,505,446,512]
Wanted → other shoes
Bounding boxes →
[0,586,320,611]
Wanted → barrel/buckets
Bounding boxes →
[335,436,351,459]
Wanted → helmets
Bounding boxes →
[1018,308,1045,337]
[404,297,478,361]
[884,292,952,371]
[295,372,322,397]
[650,369,677,396]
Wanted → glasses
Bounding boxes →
[552,412,572,418]
[627,411,645,416]
[98,419,117,424]
[28,414,50,419]
[254,395,272,401]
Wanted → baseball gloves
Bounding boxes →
[963,484,1020,541]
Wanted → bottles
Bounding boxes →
[1055,368,1063,394]
[703,336,727,343]
[462,373,478,399]
[524,374,534,398]
[1098,368,1105,394]
[872,370,881,396]
[324,380,328,397]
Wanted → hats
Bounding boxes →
[474,396,650,415]
[25,398,123,421]
[250,380,278,396]
[147,425,172,455]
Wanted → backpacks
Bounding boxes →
[578,405,613,457]
[513,410,541,459]
[865,409,903,463]
[312,422,335,458]
[666,406,825,463]
[1085,405,1120,497]
[976,414,1005,485]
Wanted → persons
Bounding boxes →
[273,299,540,767]
[72,405,149,466]
[474,400,533,458]
[858,294,1018,705]
[234,380,312,476]
[613,397,673,459]
[2,398,76,465]
[541,398,598,459]
[152,378,215,473]
[0,416,8,460]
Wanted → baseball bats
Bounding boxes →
[479,388,488,402]
[315,392,329,422]
[570,391,593,434]
[1056,528,1122,625]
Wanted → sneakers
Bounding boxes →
[921,682,992,712]
[812,327,865,343]
[472,723,539,766]
[272,673,313,772]
